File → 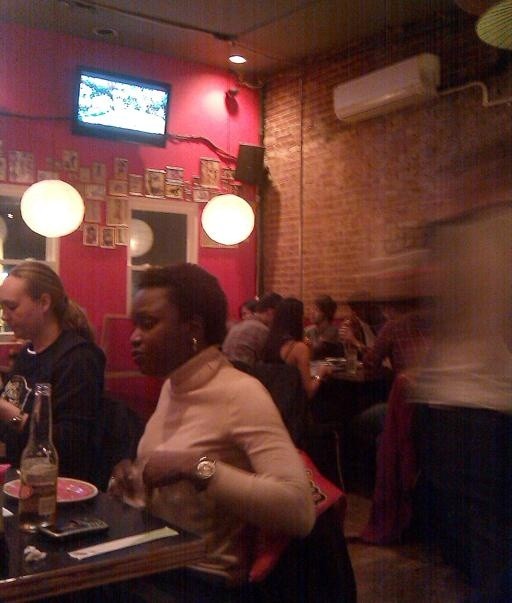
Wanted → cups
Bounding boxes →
[346,348,358,374]
[123,463,162,513]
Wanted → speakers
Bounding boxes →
[233,144,265,185]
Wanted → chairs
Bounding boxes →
[98,395,148,490]
[181,446,358,603]
[259,362,356,498]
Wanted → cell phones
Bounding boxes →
[41,519,110,541]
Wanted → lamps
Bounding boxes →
[200,26,257,245]
[131,217,155,257]
[19,0,86,236]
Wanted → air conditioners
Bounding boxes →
[329,52,441,125]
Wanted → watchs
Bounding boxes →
[8,407,27,434]
[191,456,216,492]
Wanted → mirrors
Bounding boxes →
[128,195,200,315]
[0,182,61,334]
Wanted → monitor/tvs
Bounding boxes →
[72,64,170,148]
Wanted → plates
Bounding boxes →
[3,476,100,505]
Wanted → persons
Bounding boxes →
[108,262,316,603]
[223,272,421,550]
[0,263,108,495]
[407,142,511,602]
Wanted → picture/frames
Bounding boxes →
[0,146,247,249]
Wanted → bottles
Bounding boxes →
[17,383,60,534]
